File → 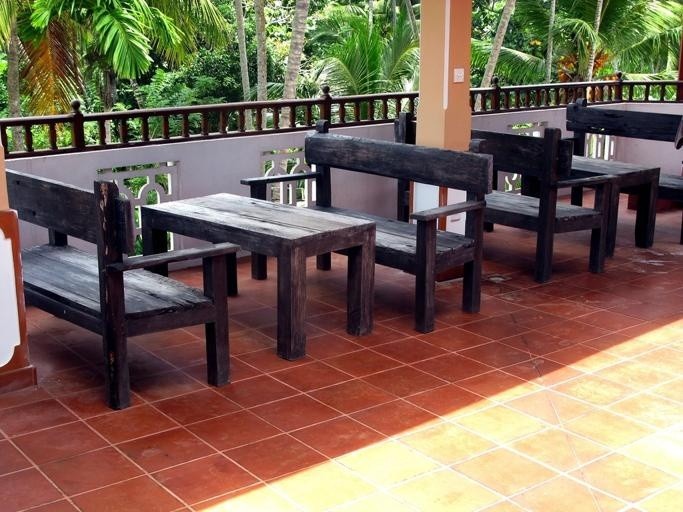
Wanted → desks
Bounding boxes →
[521,154,661,247]
[140,192,376,360]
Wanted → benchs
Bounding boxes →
[240,119,493,333]
[562,99,683,245]
[393,111,623,281]
[5,167,239,409]
